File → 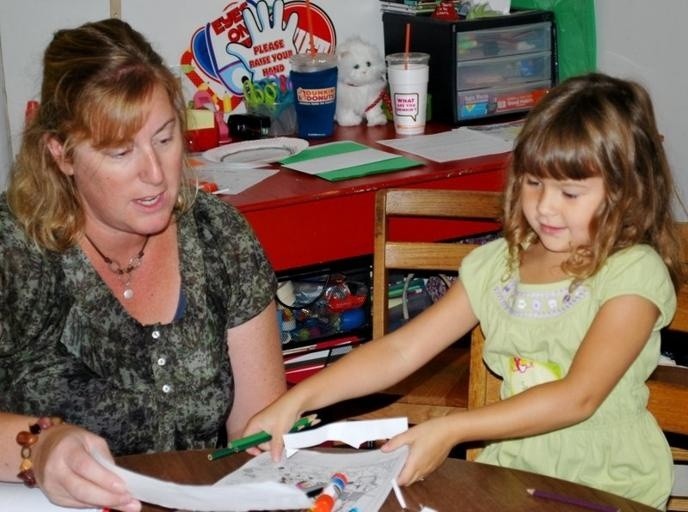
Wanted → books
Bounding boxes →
[378,0,462,17]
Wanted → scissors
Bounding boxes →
[242,75,296,135]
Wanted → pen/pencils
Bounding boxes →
[392,480,407,509]
[526,488,622,512]
[306,482,356,497]
[207,414,321,461]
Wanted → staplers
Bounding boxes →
[228,115,271,140]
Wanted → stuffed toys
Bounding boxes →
[330,35,388,128]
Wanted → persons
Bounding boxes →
[239,71,680,509]
[2,17,290,511]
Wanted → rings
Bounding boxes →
[415,474,426,484]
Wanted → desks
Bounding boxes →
[175,126,517,271]
[106,446,668,512]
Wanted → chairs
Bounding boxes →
[371,185,507,424]
[487,220,688,511]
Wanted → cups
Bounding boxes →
[289,52,339,139]
[386,51,434,136]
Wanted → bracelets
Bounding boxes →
[14,414,68,490]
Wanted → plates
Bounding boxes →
[200,134,310,170]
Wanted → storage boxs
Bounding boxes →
[383,5,559,128]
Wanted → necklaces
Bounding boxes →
[78,228,152,302]
